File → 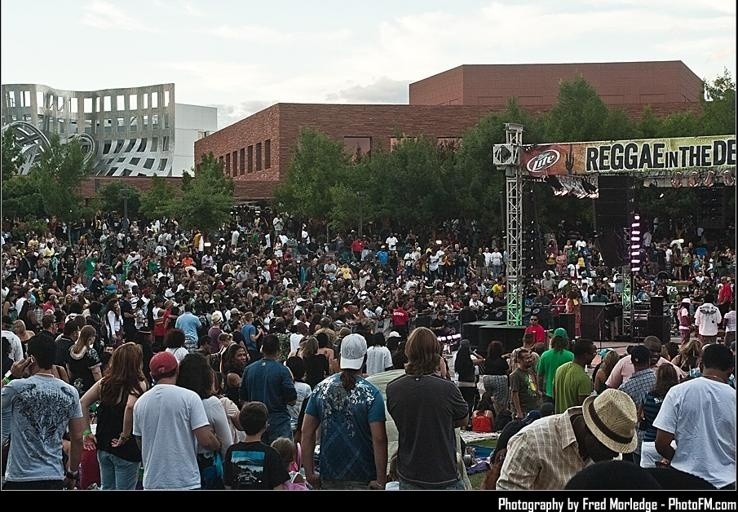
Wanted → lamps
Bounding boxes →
[542,171,602,200]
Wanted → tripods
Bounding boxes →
[595,319,606,354]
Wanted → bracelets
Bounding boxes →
[83,429,92,435]
[121,432,130,442]
[376,480,384,487]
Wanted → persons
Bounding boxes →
[2,205,738,490]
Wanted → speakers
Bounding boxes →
[696,187,728,229]
[595,176,634,225]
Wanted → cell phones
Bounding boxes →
[25,354,36,368]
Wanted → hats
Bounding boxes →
[340,333,367,370]
[582,389,638,454]
[297,298,306,303]
[150,352,177,376]
[523,410,539,423]
[13,320,35,342]
[389,332,401,338]
[211,313,221,324]
[554,327,568,340]
[682,298,690,303]
